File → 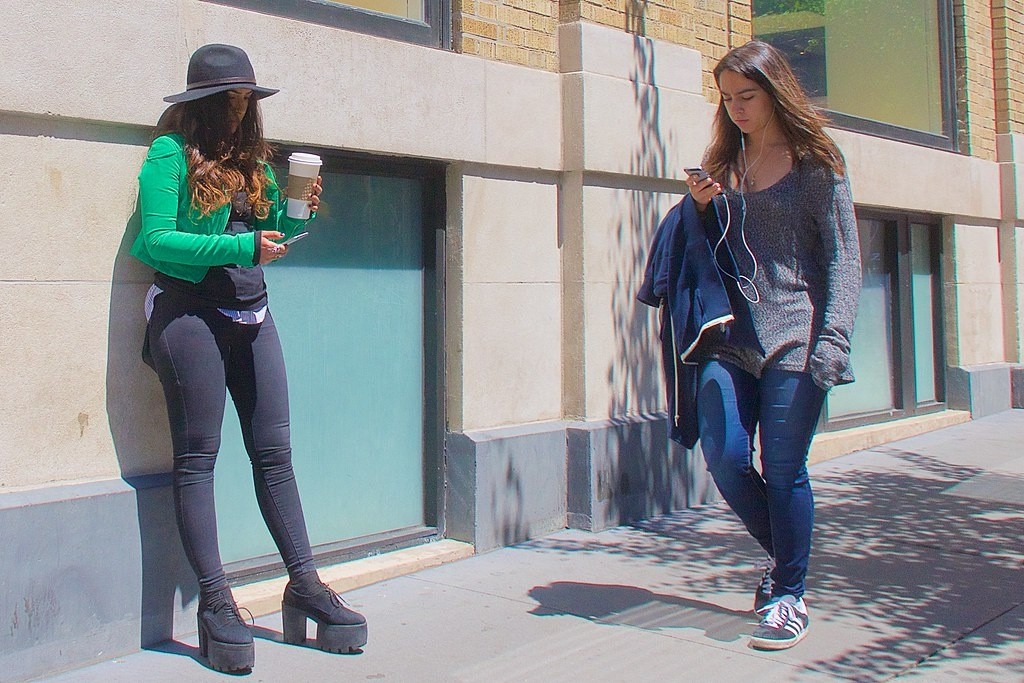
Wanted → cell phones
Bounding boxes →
[684,166,724,196]
[282,232,310,246]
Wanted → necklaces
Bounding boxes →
[748,149,772,185]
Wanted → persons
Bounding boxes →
[131,45,368,671]
[635,42,861,648]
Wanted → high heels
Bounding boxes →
[197,591,255,670]
[282,580,366,653]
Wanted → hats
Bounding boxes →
[163,43,280,102]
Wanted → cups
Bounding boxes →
[287,153,323,219]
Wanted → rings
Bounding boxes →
[272,244,280,253]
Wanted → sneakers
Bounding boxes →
[754,555,778,619]
[751,597,810,649]
